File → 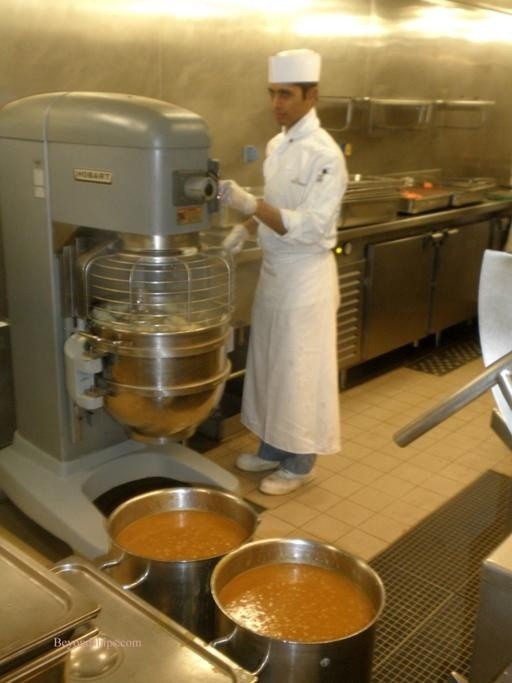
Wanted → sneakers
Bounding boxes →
[235,450,279,471]
[261,468,314,494]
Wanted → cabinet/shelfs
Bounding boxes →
[364,221,490,360]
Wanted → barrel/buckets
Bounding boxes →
[210,537,387,682]
[96,488,261,638]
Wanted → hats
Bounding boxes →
[268,48,320,85]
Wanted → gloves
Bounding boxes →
[223,223,248,253]
[223,180,257,214]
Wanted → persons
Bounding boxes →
[217,49,351,496]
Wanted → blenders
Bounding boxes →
[1,90,247,558]
